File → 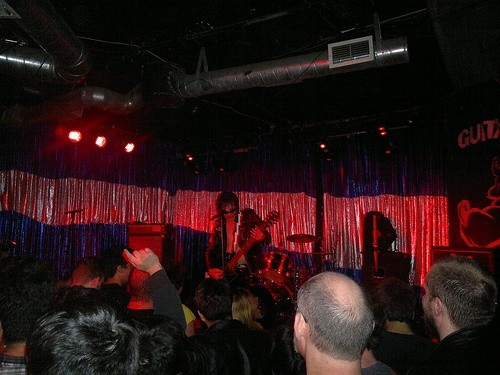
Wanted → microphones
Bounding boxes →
[211,207,229,219]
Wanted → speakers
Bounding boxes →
[125,224,173,296]
[429,245,494,278]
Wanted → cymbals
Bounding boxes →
[311,251,334,255]
[286,233,318,243]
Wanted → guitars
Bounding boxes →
[217,208,280,286]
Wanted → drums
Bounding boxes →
[258,252,310,304]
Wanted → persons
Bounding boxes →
[175,279,276,375]
[397,257,499,375]
[201,190,271,279]
[284,271,377,375]
[25,304,182,375]
[0,226,499,375]
[359,325,401,375]
